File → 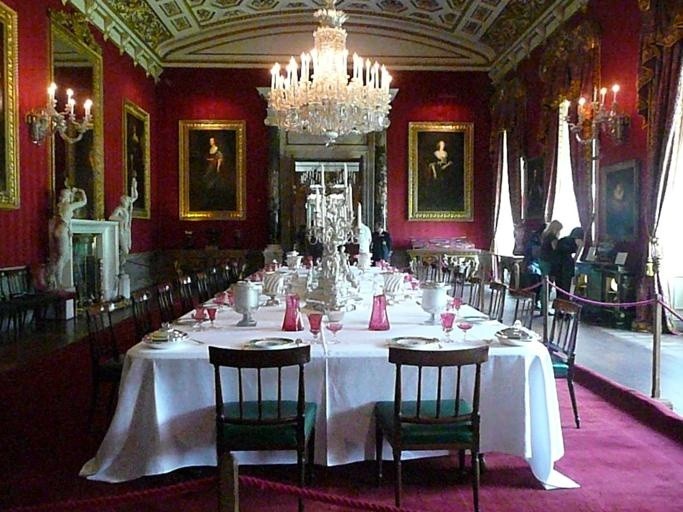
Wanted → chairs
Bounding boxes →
[376,341,491,512]
[4,222,250,436]
[408,254,588,429]
[206,343,319,509]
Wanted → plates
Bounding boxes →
[389,335,437,349]
[458,316,489,324]
[142,320,202,352]
[246,337,294,349]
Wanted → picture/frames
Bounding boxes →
[2,1,20,209]
[406,120,475,223]
[118,99,152,221]
[523,154,547,220]
[176,117,249,222]
[44,8,106,220]
[599,158,641,244]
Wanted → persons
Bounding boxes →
[370,221,392,266]
[108,178,139,276]
[521,222,549,312]
[46,186,87,289]
[551,226,585,324]
[130,124,141,179]
[527,167,543,207]
[537,220,563,317]
[194,136,229,204]
[605,174,632,233]
[309,220,322,265]
[425,137,456,206]
[294,224,308,265]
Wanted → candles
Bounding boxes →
[347,183,353,214]
[342,163,348,188]
[320,162,325,195]
[357,201,362,227]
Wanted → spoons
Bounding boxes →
[295,338,303,348]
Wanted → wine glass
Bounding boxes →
[307,312,324,345]
[195,304,219,332]
[457,321,472,343]
[214,289,235,312]
[452,298,463,314]
[326,321,344,344]
[439,312,456,344]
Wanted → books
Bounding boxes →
[584,247,596,262]
[614,252,628,265]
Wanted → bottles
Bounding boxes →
[367,283,391,330]
[118,274,130,299]
[281,292,305,331]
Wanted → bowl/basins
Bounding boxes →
[494,331,543,346]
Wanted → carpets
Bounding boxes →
[3,299,682,512]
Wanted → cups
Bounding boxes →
[262,272,284,305]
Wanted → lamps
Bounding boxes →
[26,81,94,146]
[256,0,402,142]
[563,80,630,148]
